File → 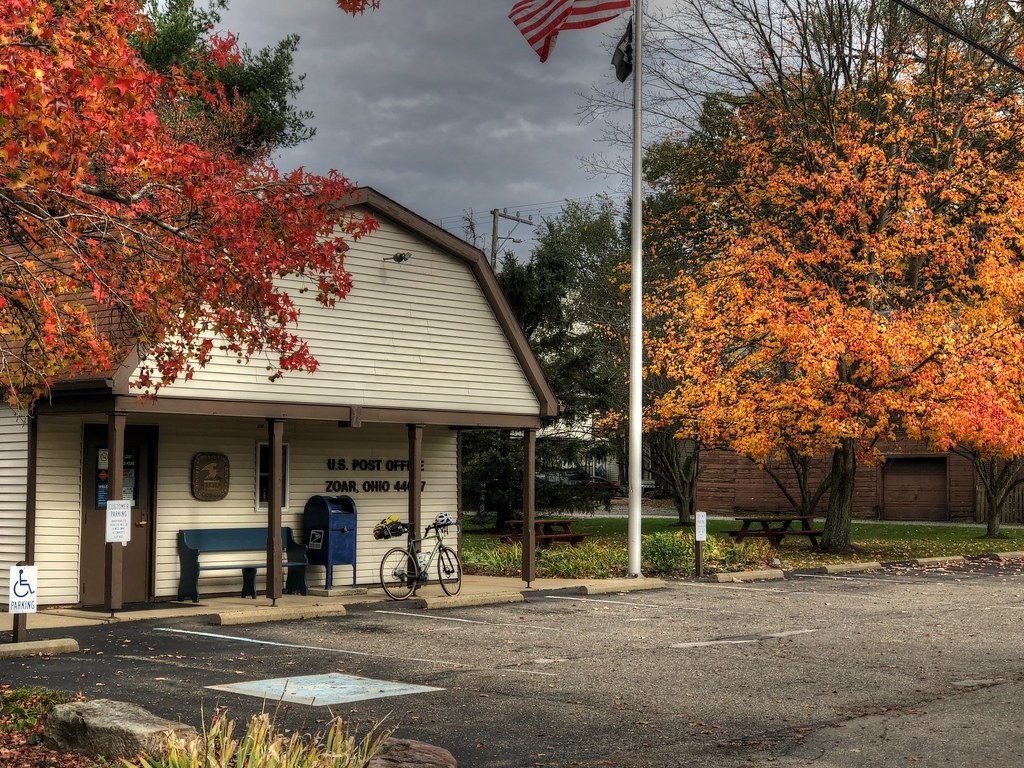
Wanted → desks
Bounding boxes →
[734,515,819,550]
[504,519,578,550]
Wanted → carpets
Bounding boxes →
[84,599,207,613]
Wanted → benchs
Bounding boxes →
[496,532,594,545]
[177,526,310,604]
[720,529,825,539]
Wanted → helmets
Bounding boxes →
[435,512,453,525]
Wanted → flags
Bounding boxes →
[507,0,635,63]
[611,15,633,84]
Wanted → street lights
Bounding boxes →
[491,236,522,276]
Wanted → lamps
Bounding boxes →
[385,251,411,263]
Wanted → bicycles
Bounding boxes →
[380,520,462,601]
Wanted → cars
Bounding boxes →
[472,475,555,512]
[576,476,624,500]
